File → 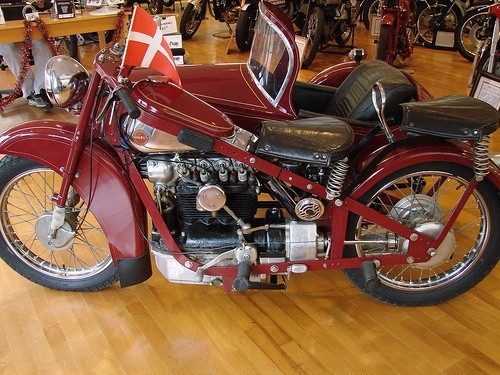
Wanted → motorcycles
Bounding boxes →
[0,0,500,308]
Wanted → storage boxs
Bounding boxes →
[160,14,184,65]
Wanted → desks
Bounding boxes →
[0,9,132,55]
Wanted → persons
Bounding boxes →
[0,0,55,112]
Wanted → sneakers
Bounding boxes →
[28,94,48,108]
[44,101,56,112]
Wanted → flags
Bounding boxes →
[122,5,183,91]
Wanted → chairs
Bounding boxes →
[298,59,415,121]
